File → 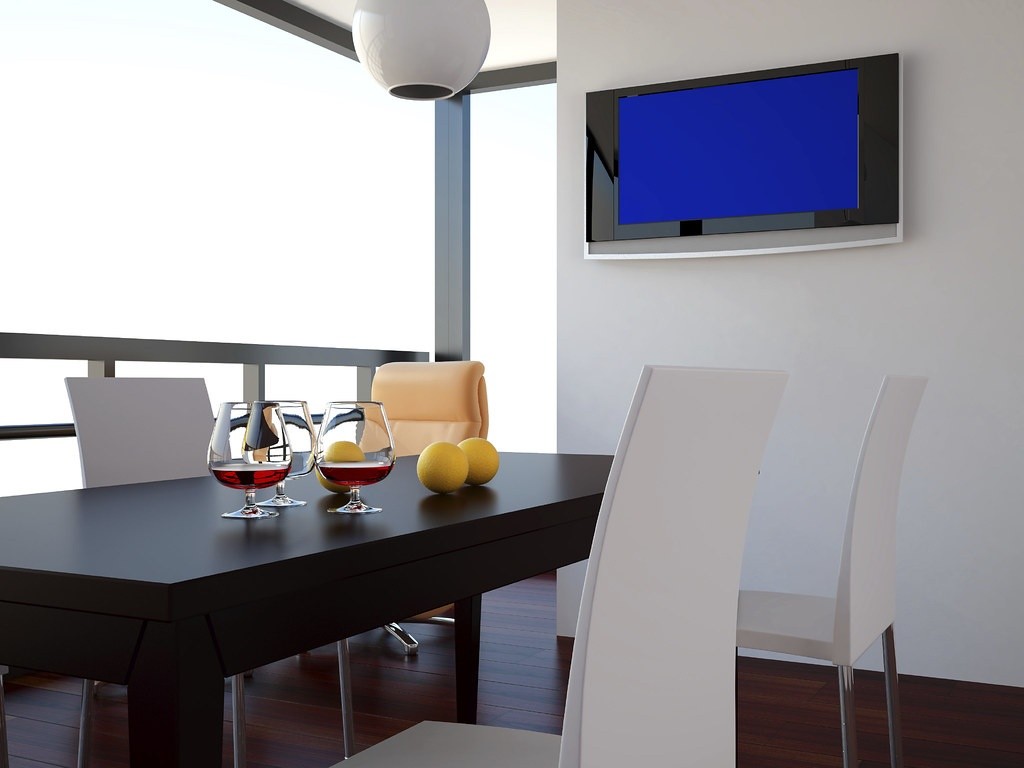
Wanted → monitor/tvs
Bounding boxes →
[585,50,902,242]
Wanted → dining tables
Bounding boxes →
[1,448,639,768]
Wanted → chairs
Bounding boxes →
[354,358,486,466]
[322,356,800,768]
[64,375,228,491]
[743,377,936,768]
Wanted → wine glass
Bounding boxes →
[242,398,318,507]
[206,400,293,521]
[313,401,396,513]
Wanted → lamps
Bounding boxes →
[347,1,497,104]
